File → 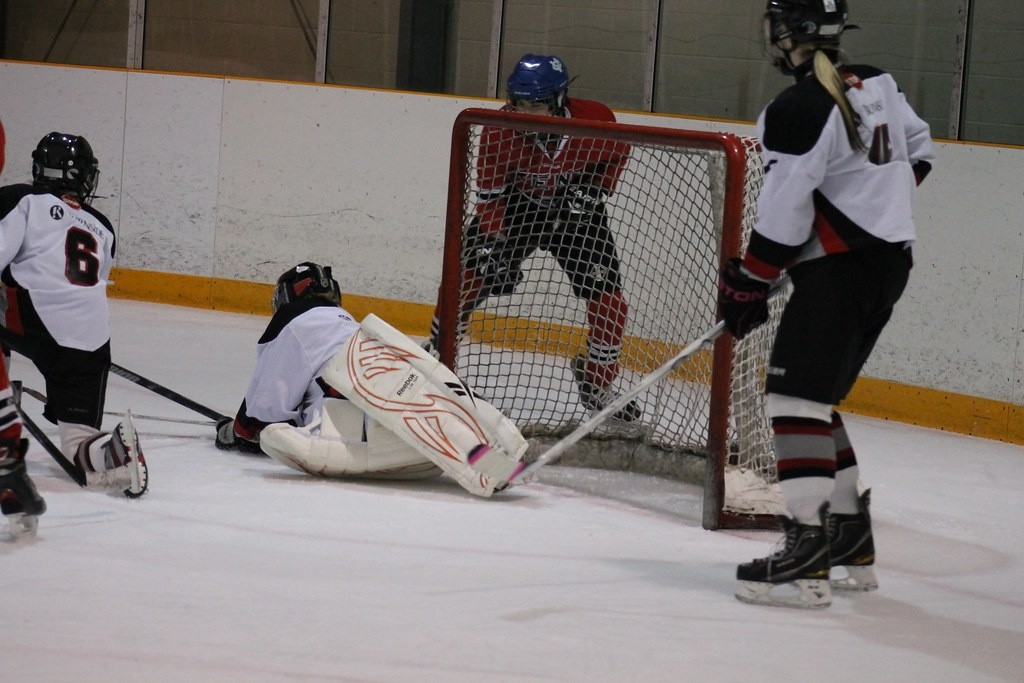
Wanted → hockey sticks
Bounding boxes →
[433,222,559,355]
[5,379,141,496]
[441,269,795,487]
[106,360,236,428]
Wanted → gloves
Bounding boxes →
[474,238,524,295]
[215,418,247,452]
[716,258,771,340]
[553,182,604,229]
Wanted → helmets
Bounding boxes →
[759,0,861,75]
[271,262,342,314]
[31,132,100,207]
[505,54,580,116]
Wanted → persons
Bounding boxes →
[215,262,529,498]
[717,0,936,610]
[0,131,149,542]
[421,53,645,428]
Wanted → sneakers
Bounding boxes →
[826,488,878,592]
[570,354,641,427]
[422,340,440,361]
[735,501,833,609]
[0,438,46,543]
[102,408,148,499]
